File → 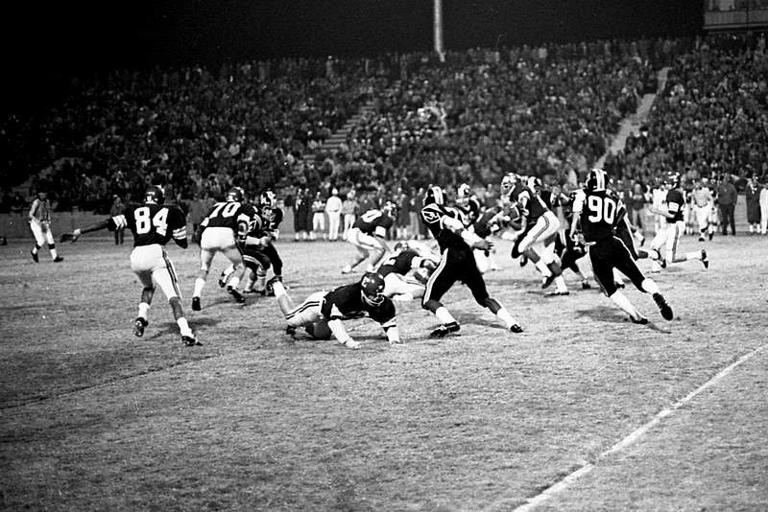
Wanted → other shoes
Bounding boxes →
[341,264,356,275]
[392,294,415,304]
[365,266,374,271]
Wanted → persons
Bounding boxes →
[1,38,768,349]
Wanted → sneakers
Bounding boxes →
[653,293,674,321]
[652,247,667,269]
[631,314,649,324]
[511,324,522,334]
[134,269,312,345]
[700,249,708,268]
[428,321,461,338]
[520,255,627,297]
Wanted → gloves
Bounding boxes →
[58,229,79,245]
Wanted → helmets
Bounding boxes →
[359,272,385,307]
[226,187,245,201]
[143,184,165,205]
[260,190,277,209]
[382,169,681,222]
[395,241,409,253]
